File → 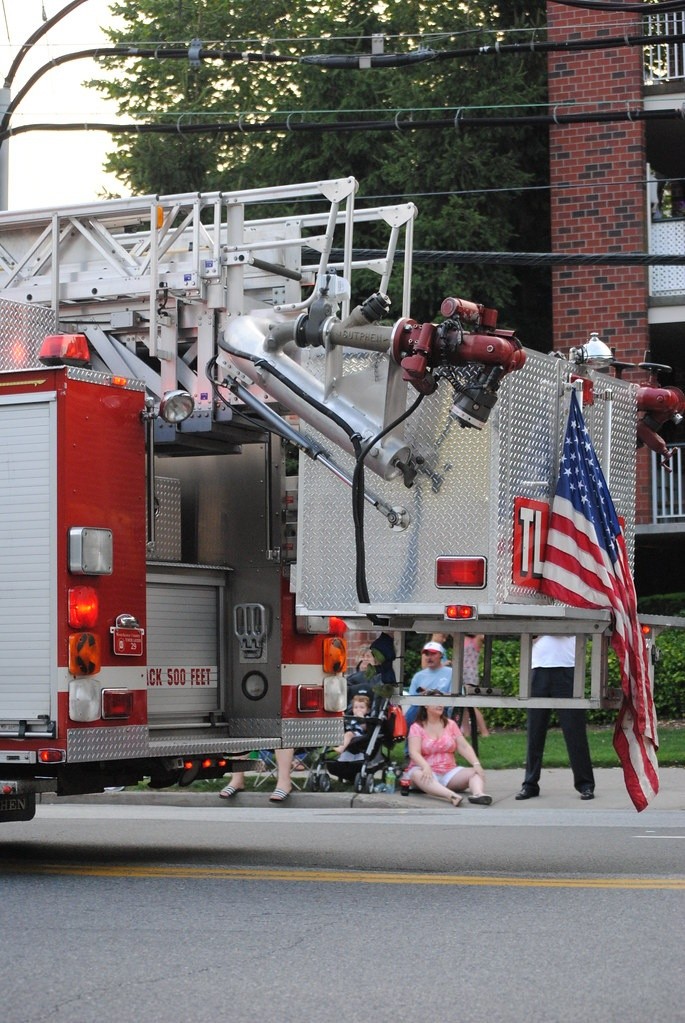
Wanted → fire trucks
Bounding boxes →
[0,174,685,822]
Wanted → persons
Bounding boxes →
[515,636,596,800]
[219,631,493,806]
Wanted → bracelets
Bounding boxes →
[474,763,480,765]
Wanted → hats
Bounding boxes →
[421,641,445,656]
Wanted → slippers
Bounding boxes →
[219,786,244,799]
[269,788,292,802]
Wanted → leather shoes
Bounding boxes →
[581,789,594,800]
[515,789,540,800]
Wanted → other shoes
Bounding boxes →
[468,794,492,805]
[258,766,266,772]
[291,759,305,771]
[450,794,463,806]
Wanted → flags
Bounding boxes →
[539,390,661,814]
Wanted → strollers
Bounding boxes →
[310,670,406,794]
